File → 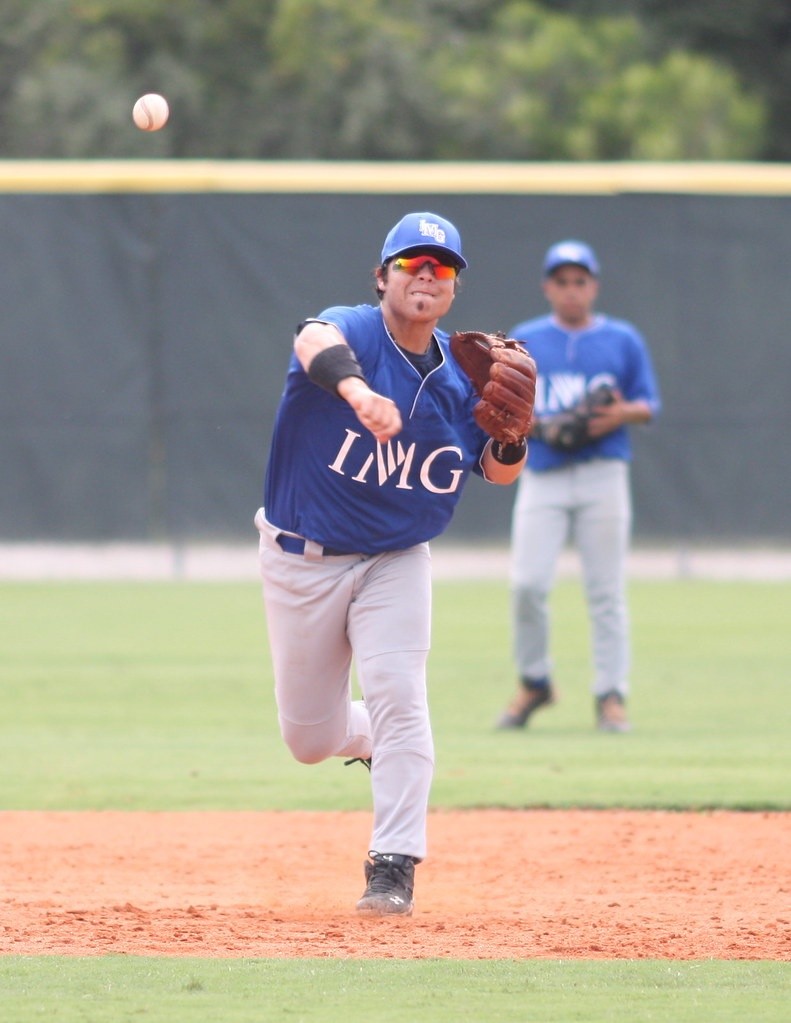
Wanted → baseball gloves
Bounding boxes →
[449,330,537,447]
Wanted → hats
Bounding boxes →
[380,212,468,272]
[542,238,601,278]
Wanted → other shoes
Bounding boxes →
[596,689,626,732]
[495,678,554,730]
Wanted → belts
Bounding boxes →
[275,534,354,556]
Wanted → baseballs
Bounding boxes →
[132,93,170,132]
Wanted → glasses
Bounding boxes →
[385,252,457,282]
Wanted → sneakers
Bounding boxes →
[344,757,371,770]
[355,850,416,918]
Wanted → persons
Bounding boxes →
[250,212,538,921]
[492,238,661,733]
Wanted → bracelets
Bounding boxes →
[491,437,528,465]
[308,345,367,402]
